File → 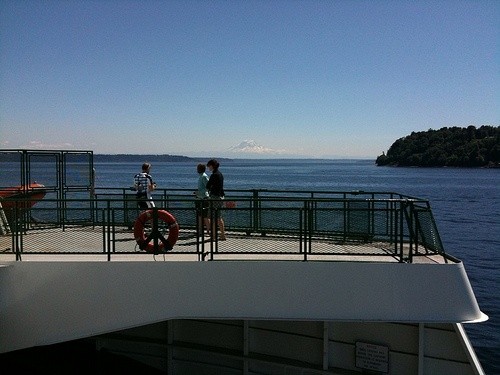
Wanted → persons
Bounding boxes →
[206,159,227,243]
[194,163,212,238]
[133,163,157,212]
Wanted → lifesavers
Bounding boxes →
[134,211,178,252]
